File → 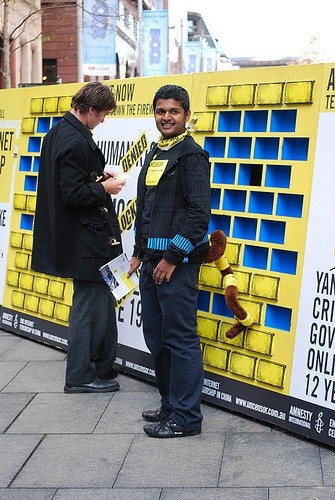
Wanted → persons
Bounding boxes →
[126,85,211,438]
[31,81,126,394]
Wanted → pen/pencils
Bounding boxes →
[106,171,126,186]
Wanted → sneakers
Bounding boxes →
[143,417,202,438]
[141,409,166,422]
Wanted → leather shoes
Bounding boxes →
[96,367,118,380]
[64,376,120,393]
